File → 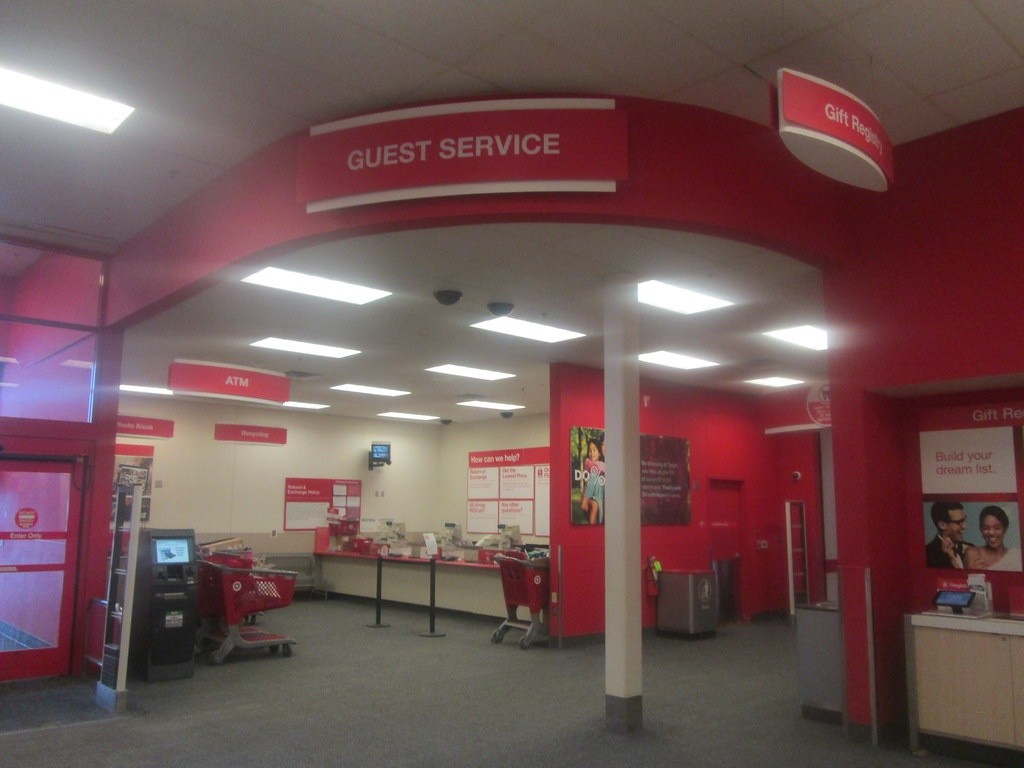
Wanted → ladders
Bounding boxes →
[783,500,812,616]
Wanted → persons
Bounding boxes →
[924,502,976,569]
[580,439,605,524]
[941,506,1022,571]
[581,432,605,523]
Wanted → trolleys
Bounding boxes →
[491,548,549,650]
[196,560,300,665]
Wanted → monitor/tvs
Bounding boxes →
[372,442,392,463]
[148,534,192,566]
[934,588,975,608]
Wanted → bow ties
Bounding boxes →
[952,543,963,558]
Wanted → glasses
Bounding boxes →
[939,514,966,524]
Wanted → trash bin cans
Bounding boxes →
[794,603,846,725]
[655,570,720,639]
